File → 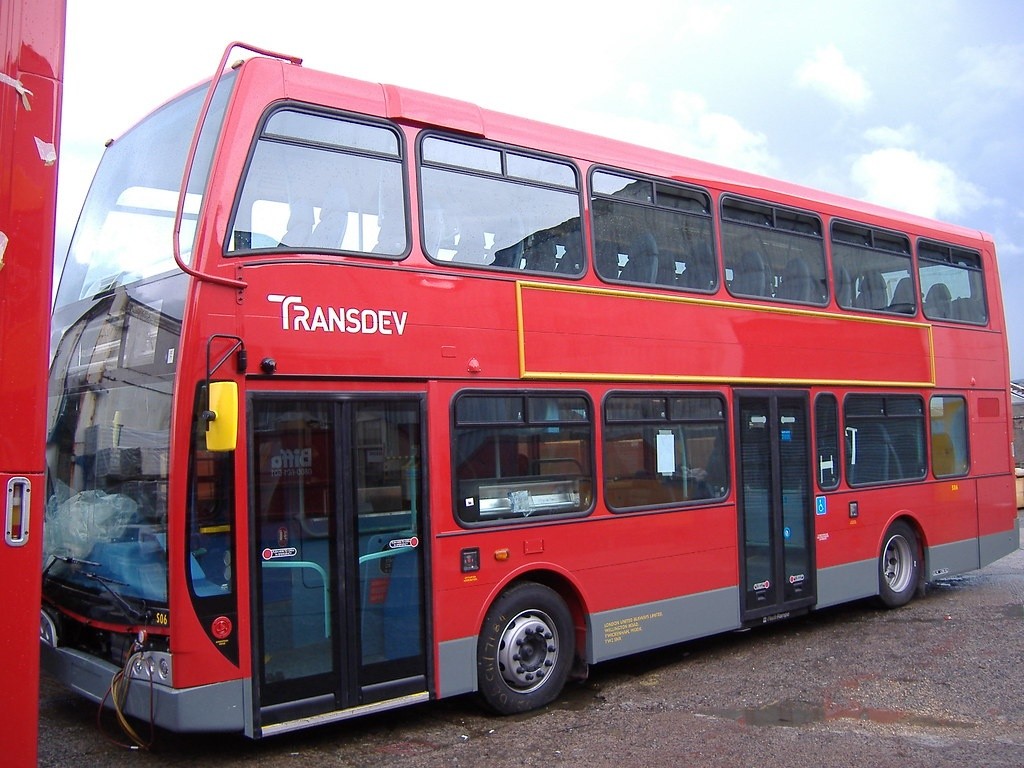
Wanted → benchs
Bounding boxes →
[516,432,715,510]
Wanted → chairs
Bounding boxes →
[276,184,954,319]
[708,406,924,488]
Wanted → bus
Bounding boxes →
[40,40,1020,750]
